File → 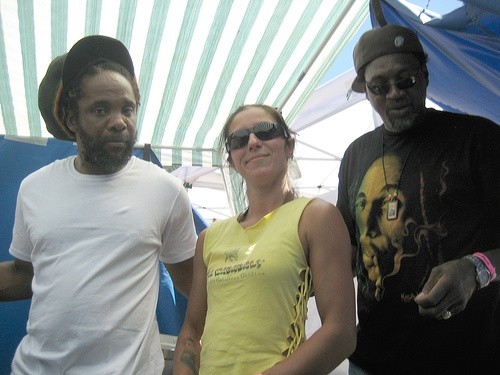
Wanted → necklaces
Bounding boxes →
[382,107,430,220]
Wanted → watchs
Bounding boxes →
[464,254,491,290]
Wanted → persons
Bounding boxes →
[0,34,200,375]
[172,105,357,375]
[337,24,500,375]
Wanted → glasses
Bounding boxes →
[365,64,420,95]
[225,122,284,151]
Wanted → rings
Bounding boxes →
[442,312,452,320]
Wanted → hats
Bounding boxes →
[351,24,424,92]
[37,35,134,142]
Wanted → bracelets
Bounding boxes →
[472,252,497,283]
